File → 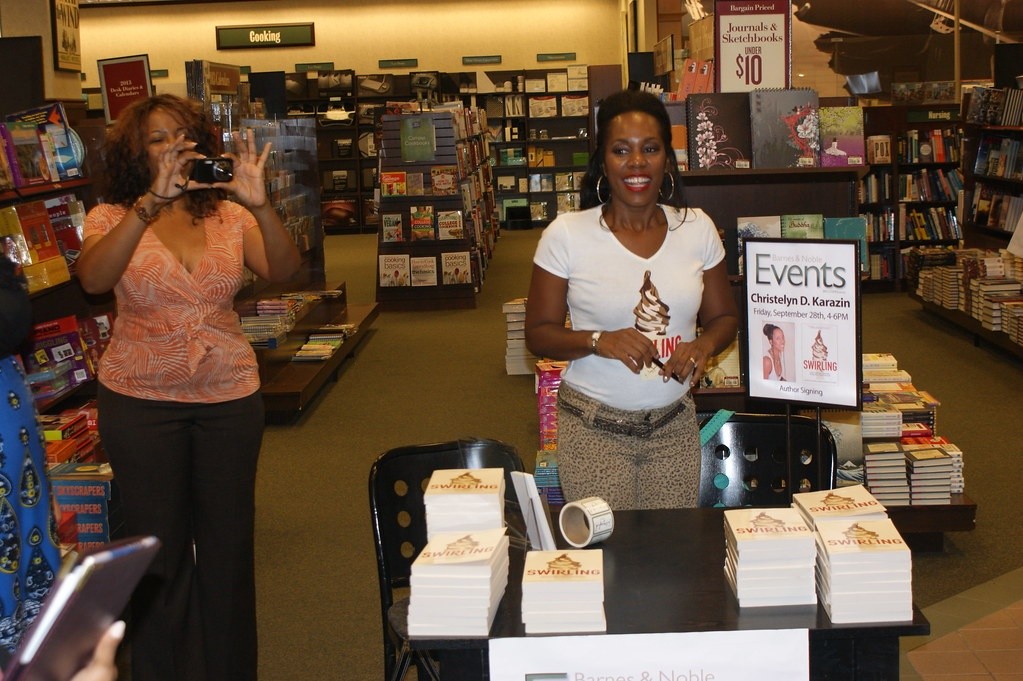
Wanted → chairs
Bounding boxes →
[369,438,536,681]
[691,412,838,508]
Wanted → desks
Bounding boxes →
[408,506,930,681]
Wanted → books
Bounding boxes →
[240,291,358,359]
[686,85,870,275]
[407,468,606,636]
[325,100,500,286]
[503,297,566,503]
[961,85,1023,232]
[906,248,1023,347]
[857,129,963,279]
[798,354,965,505]
[6,533,161,680]
[723,485,913,623]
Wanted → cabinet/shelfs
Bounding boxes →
[258,66,595,234]
[371,113,497,294]
[862,108,962,289]
[962,94,1023,259]
[0,110,124,477]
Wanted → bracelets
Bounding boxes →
[591,330,606,356]
[134,196,162,225]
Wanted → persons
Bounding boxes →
[73,93,301,681]
[0,252,62,664]
[0,102,114,464]
[71,619,126,680]
[763,323,787,381]
[524,90,739,510]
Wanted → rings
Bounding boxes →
[690,357,695,364]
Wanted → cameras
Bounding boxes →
[195,158,233,183]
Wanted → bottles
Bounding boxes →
[517,76,524,92]
[540,130,548,139]
[529,129,538,139]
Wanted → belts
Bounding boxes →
[557,389,691,437]
